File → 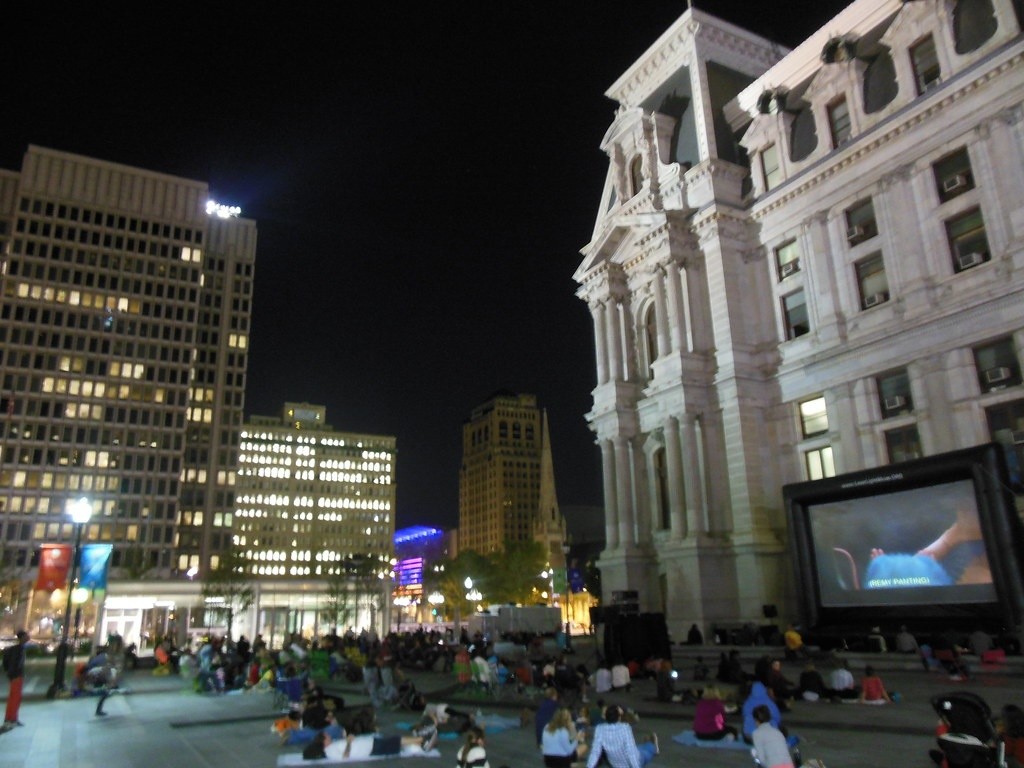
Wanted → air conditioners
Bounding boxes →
[987,367,1010,383]
[959,252,983,269]
[1011,431,1024,445]
[783,264,797,276]
[925,77,941,93]
[838,135,852,149]
[865,294,885,308]
[885,395,906,410]
[846,224,864,242]
[943,176,966,192]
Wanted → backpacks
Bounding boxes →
[2,643,18,671]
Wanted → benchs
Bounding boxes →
[671,639,820,659]
[832,649,1024,673]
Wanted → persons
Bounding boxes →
[72,635,127,716]
[154,631,276,695]
[835,494,992,590]
[271,620,1001,768]
[2,629,29,727]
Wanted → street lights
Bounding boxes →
[45,496,94,699]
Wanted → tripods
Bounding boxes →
[585,624,603,668]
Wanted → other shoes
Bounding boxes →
[4,721,24,727]
[652,731,660,754]
[95,711,107,715]
[419,729,438,752]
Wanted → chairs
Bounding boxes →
[273,678,307,713]
[452,661,520,702]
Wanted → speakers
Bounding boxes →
[763,605,778,619]
[760,625,779,646]
[589,606,603,625]
